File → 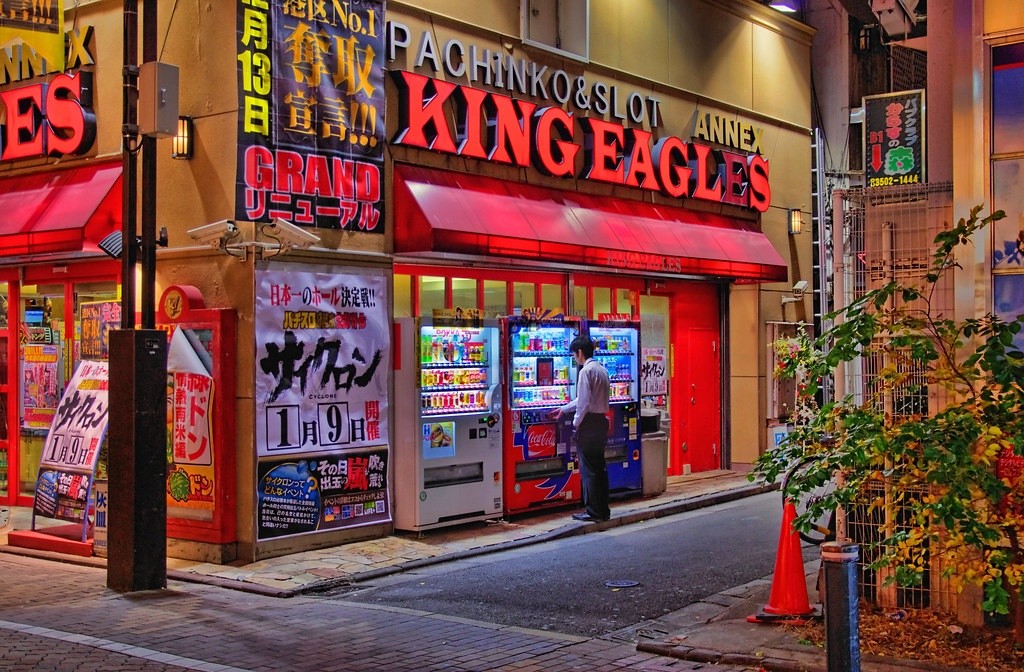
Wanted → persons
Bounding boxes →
[548,336,610,522]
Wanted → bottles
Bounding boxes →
[421,367,487,390]
[421,334,485,365]
[513,386,571,403]
[513,330,569,352]
[421,390,486,411]
[553,366,568,381]
[513,363,535,382]
[598,358,629,380]
[592,336,628,353]
[609,383,632,399]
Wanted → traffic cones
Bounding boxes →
[747,499,828,622]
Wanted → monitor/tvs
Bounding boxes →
[24,311,44,328]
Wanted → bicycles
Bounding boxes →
[781,433,842,544]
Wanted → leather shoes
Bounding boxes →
[571,511,610,521]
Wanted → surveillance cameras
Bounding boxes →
[187,219,237,244]
[273,217,321,250]
[792,280,809,296]
